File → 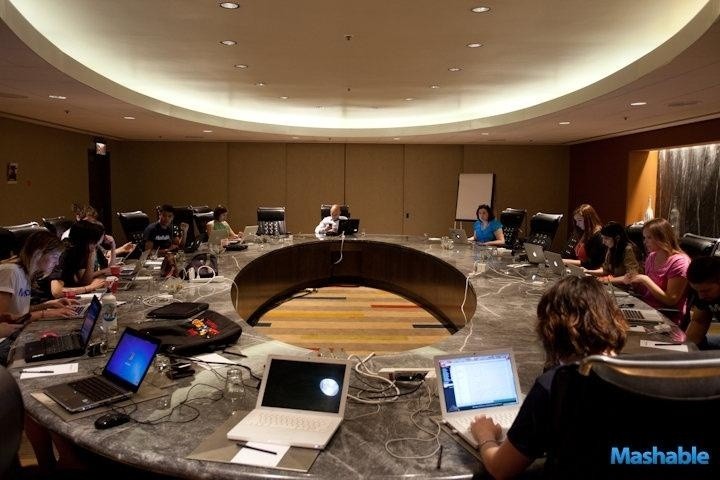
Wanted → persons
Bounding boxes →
[0,322,23,339]
[684,253,720,351]
[140,203,179,253]
[0,229,80,324]
[205,204,244,241]
[59,199,134,265]
[621,218,691,325]
[90,220,116,279]
[467,204,505,245]
[560,204,609,270]
[580,219,640,293]
[469,274,629,480]
[44,220,113,298]
[314,204,349,234]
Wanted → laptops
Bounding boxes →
[523,242,545,263]
[24,295,102,363]
[145,302,209,320]
[607,290,629,296]
[64,291,106,319]
[620,307,665,323]
[171,233,203,253]
[41,326,162,413]
[449,229,473,245]
[227,353,352,451]
[432,348,525,450]
[232,224,259,244]
[120,249,150,276]
[115,245,135,265]
[96,268,140,291]
[544,251,583,276]
[326,219,360,236]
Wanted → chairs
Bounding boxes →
[116,210,150,244]
[190,204,213,243]
[499,207,524,247]
[529,212,564,252]
[628,234,720,332]
[627,222,675,264]
[42,216,69,240]
[579,351,720,479]
[158,205,197,247]
[256,205,286,236]
[320,204,351,221]
[0,364,37,480]
[0,221,50,256]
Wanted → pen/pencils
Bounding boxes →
[19,370,54,373]
[655,344,681,345]
[437,445,443,469]
[237,443,277,455]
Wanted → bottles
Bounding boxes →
[101,289,116,333]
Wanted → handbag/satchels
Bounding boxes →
[509,239,525,248]
[179,253,218,279]
[141,311,242,357]
[1,332,20,367]
[161,255,178,277]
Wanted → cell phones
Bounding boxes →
[329,224,332,229]
[171,362,191,370]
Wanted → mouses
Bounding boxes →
[94,412,129,430]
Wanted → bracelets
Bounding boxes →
[480,437,499,450]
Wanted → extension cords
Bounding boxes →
[468,268,483,278]
[378,367,435,393]
[189,276,224,283]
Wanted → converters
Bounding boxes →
[477,263,482,272]
[158,293,173,300]
[424,370,437,386]
[188,268,195,279]
[481,264,489,272]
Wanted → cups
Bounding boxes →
[154,359,170,409]
[226,368,244,415]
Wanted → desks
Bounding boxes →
[7,234,700,480]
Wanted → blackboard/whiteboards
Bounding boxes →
[455,173,495,221]
